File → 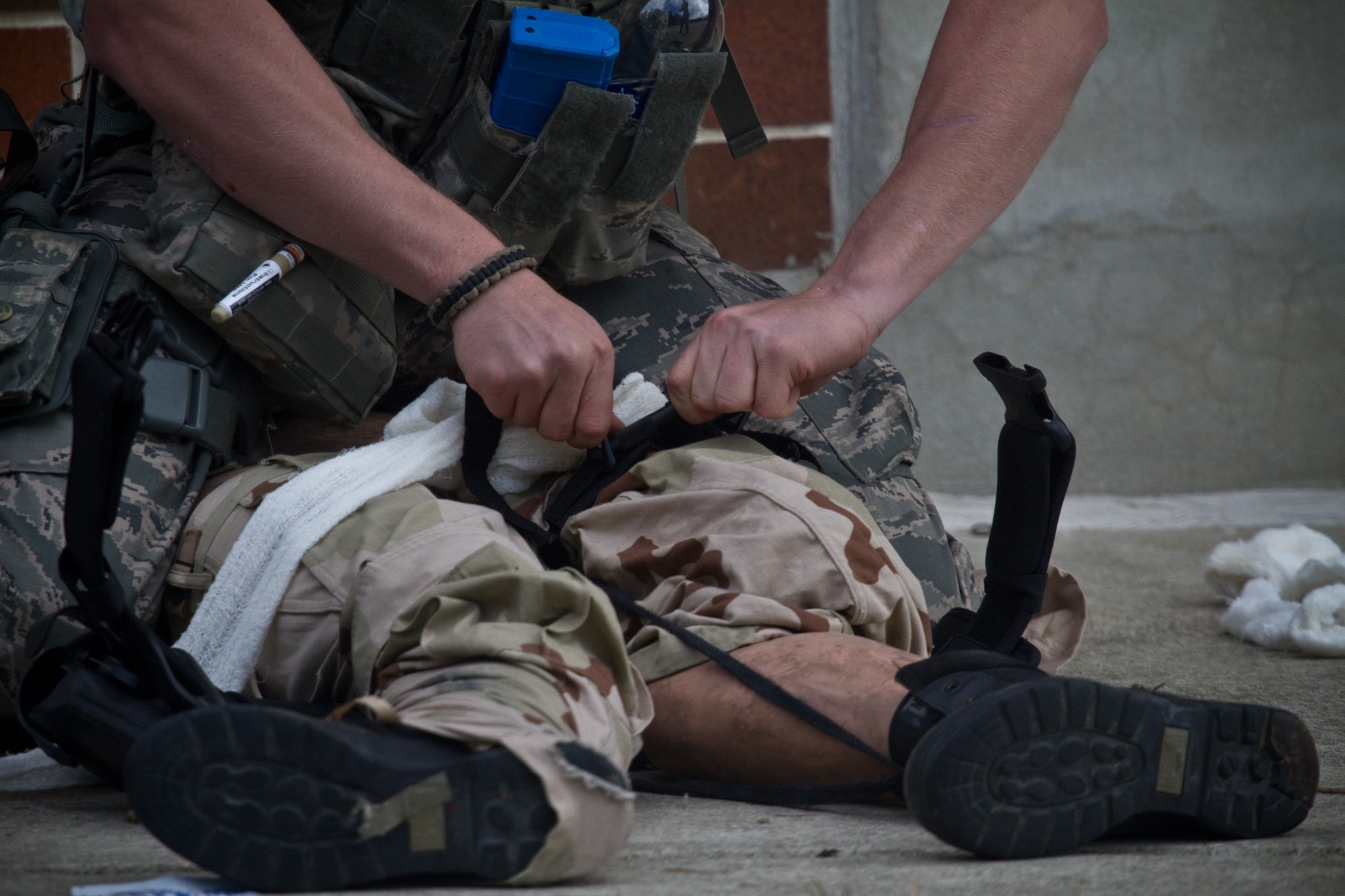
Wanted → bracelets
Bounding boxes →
[425,242,538,332]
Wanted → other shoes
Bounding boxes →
[889,650,1320,858]
[124,704,557,891]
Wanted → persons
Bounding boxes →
[1,0,1319,889]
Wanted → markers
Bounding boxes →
[211,239,306,323]
